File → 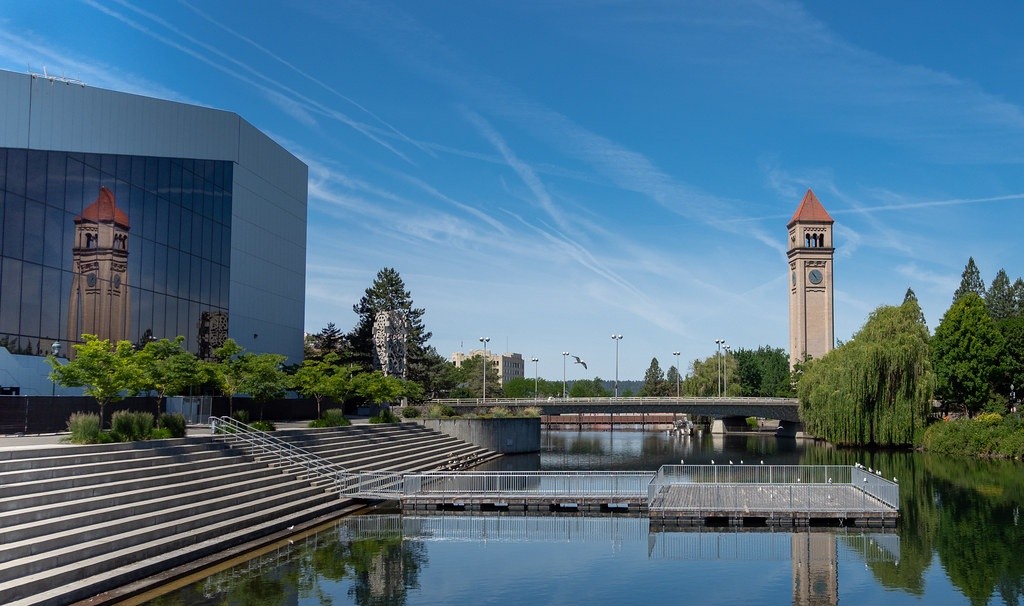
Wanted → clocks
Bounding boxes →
[114,274,121,289]
[87,273,97,286]
[813,582,826,594]
[792,271,796,286]
[809,269,823,283]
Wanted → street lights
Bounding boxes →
[532,358,539,400]
[612,334,623,397]
[480,337,490,404]
[723,345,730,398]
[562,352,569,399]
[715,339,725,398]
[673,351,681,397]
[49,341,62,395]
